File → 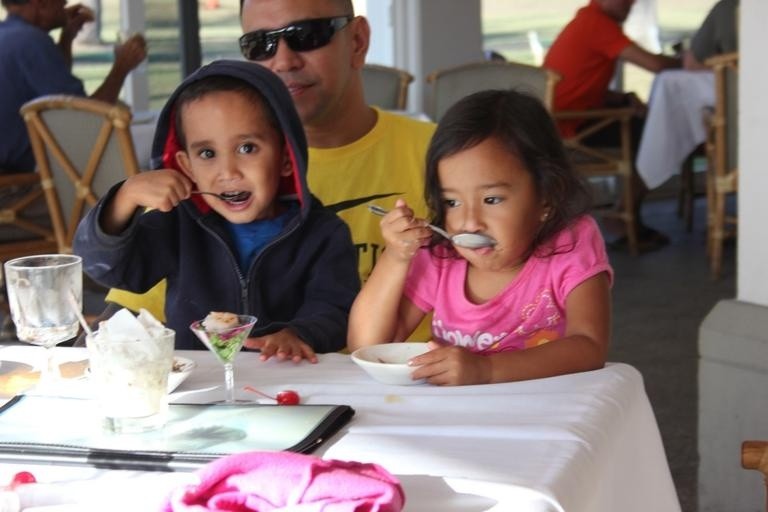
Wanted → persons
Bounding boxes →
[70,59,362,368]
[0,0,146,247]
[537,2,680,252]
[345,86,616,392]
[71,0,439,356]
[684,1,740,179]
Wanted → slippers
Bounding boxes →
[606,228,663,253]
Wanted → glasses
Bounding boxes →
[238,15,355,61]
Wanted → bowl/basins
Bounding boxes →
[83,356,196,395]
[349,340,436,387]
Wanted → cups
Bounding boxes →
[84,325,177,434]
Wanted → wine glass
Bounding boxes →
[3,253,87,399]
[188,313,260,406]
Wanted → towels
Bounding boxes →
[159,447,406,512]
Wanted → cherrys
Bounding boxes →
[244,386,299,405]
[13,472,35,483]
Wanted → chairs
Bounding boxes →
[423,49,740,282]
[19,96,142,256]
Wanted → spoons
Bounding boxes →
[190,190,252,204]
[366,204,499,250]
